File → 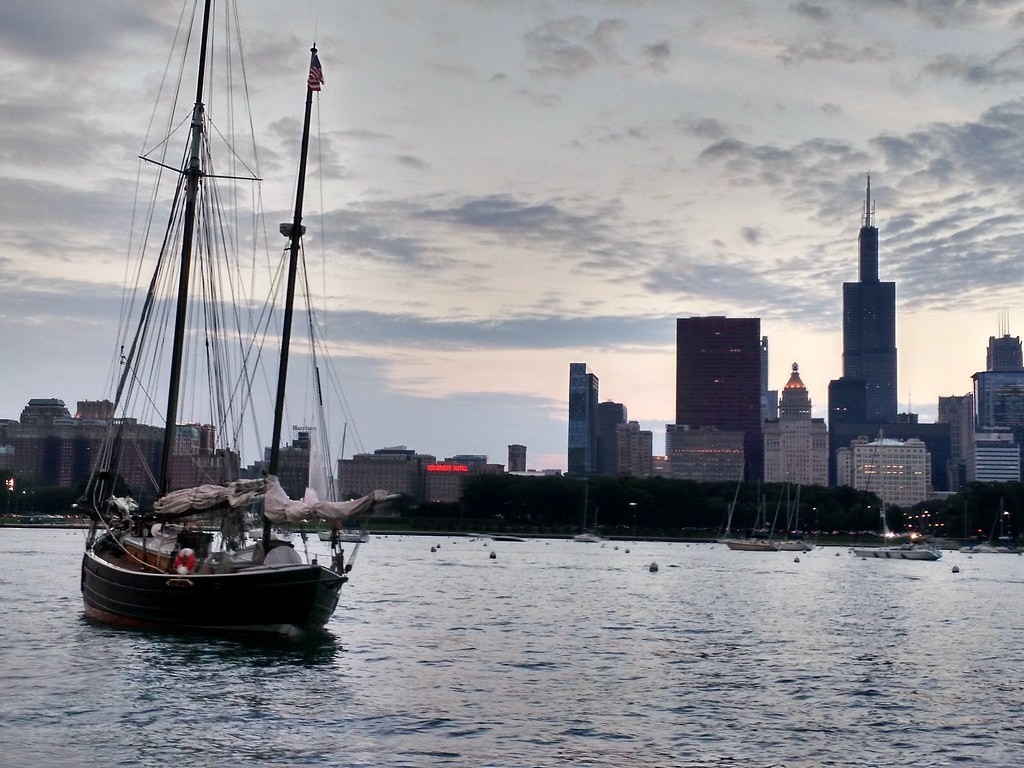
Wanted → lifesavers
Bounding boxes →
[176,548,196,575]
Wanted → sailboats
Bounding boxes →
[76,1,405,630]
[988,496,1024,553]
[851,427,944,561]
[723,397,812,551]
[316,421,369,543]
[573,483,600,542]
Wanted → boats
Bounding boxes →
[491,535,525,542]
